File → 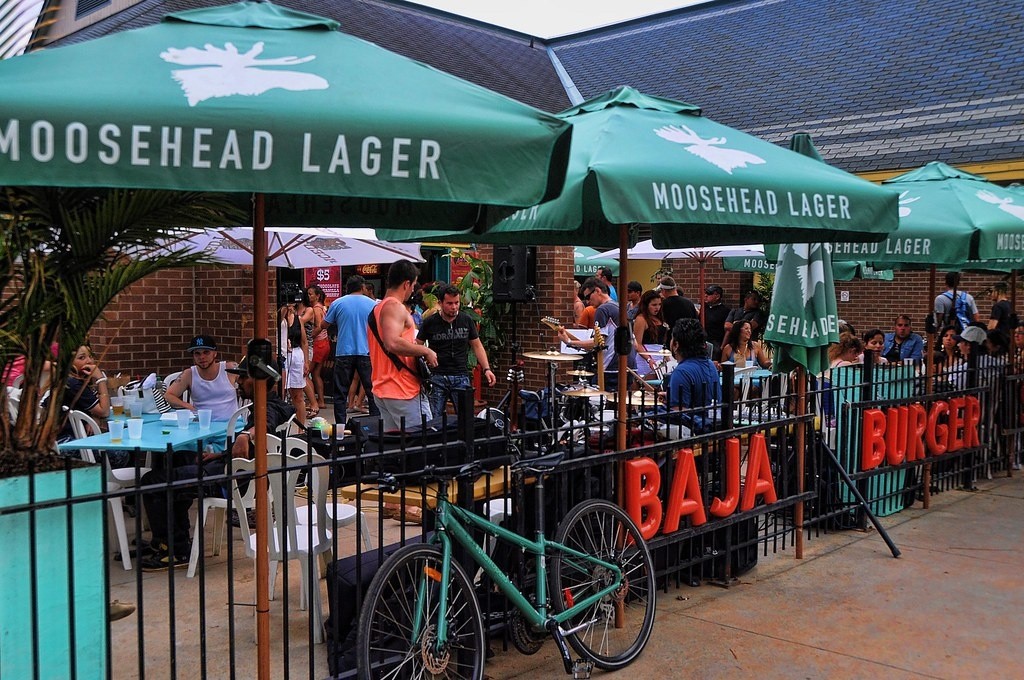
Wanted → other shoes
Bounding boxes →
[306,408,320,419]
[379,505,398,519]
[392,508,423,524]
[348,405,361,414]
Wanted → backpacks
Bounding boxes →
[942,290,975,332]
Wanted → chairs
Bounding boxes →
[653,360,1003,550]
[6,380,374,650]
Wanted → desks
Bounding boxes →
[342,463,563,541]
[56,419,248,562]
[637,378,770,391]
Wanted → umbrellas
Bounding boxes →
[765,162,1024,507]
[763,128,840,567]
[377,83,900,628]
[0,1,575,679]
[926,183,1024,315]
[586,239,765,329]
[109,226,432,265]
[573,245,620,276]
[720,256,894,282]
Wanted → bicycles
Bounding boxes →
[356,453,657,680]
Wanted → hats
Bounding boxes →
[628,281,642,292]
[186,335,217,352]
[952,326,987,346]
[704,285,723,297]
[225,352,279,392]
[655,283,676,290]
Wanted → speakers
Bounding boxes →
[493,244,537,303]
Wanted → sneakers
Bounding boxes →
[136,537,194,572]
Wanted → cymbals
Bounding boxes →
[560,386,608,396]
[637,349,672,356]
[608,390,663,405]
[522,351,583,361]
[566,371,594,376]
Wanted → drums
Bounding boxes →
[593,410,627,422]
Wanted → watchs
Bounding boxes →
[241,430,252,441]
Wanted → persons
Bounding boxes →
[277,257,497,429]
[815,272,1024,419]
[571,264,775,441]
[163,334,244,466]
[114,353,299,571]
[57,339,113,450]
[2,339,60,428]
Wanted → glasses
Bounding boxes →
[989,288,999,293]
[584,289,595,300]
[943,334,954,337]
[745,290,757,297]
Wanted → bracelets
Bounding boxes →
[95,377,108,384]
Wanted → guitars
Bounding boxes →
[541,316,597,371]
[506,368,526,438]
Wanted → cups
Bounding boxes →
[904,359,913,365]
[122,396,135,413]
[735,368,742,378]
[321,425,329,439]
[127,420,143,439]
[130,403,142,418]
[336,424,345,440]
[176,410,190,429]
[108,421,124,439]
[198,410,212,429]
[746,361,753,375]
[111,397,123,415]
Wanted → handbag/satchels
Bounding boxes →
[414,355,434,379]
